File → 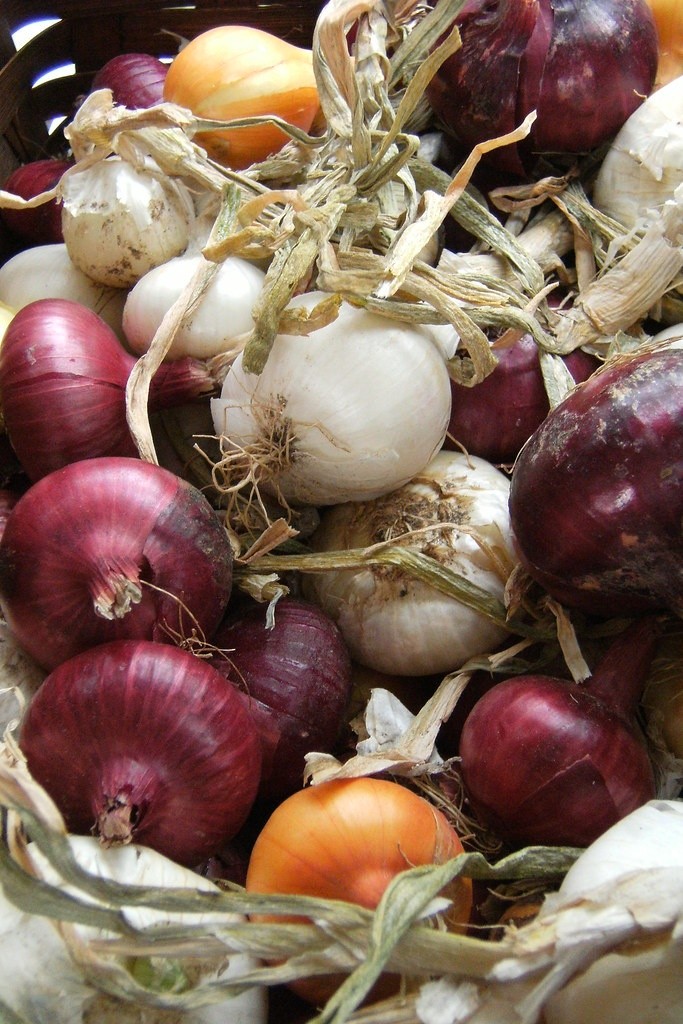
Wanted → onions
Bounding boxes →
[0,0,683,1008]
